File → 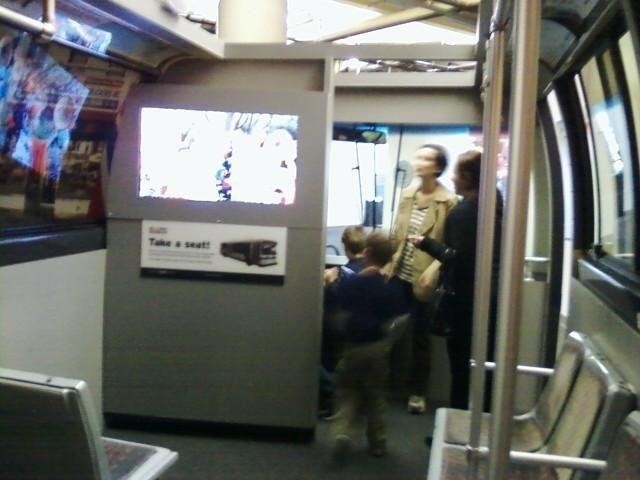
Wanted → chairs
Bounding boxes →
[422,333,640,480]
[0,365,179,480]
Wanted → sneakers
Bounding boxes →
[425,436,432,446]
[331,434,386,456]
[407,394,426,413]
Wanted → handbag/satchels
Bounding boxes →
[429,248,465,337]
